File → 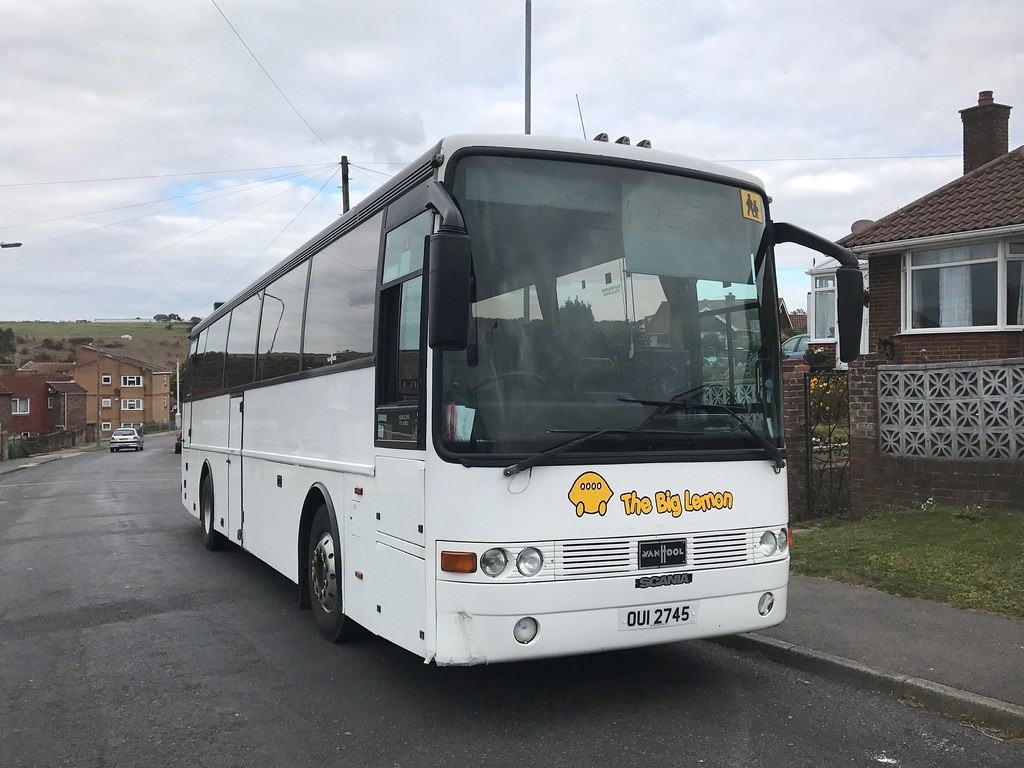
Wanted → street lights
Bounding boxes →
[95,334,133,446]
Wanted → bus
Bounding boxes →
[178,131,863,667]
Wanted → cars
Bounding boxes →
[175,431,182,454]
[109,428,145,450]
[733,333,810,374]
